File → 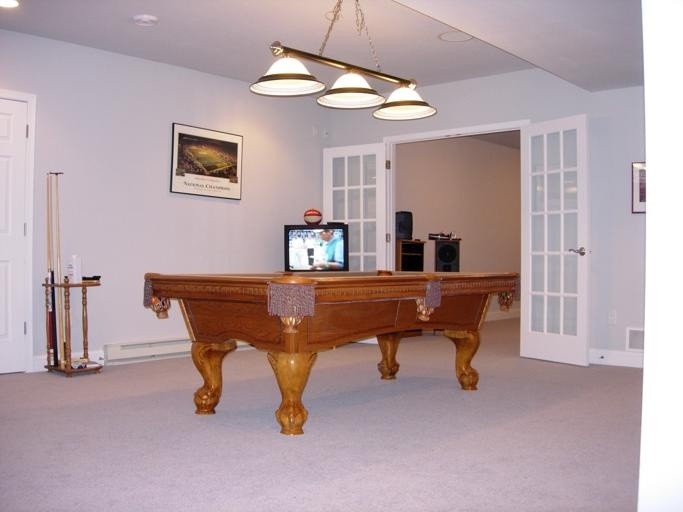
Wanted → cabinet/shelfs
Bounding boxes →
[396,240,426,337]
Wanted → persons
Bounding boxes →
[306,229,344,270]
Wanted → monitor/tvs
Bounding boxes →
[285,224,349,271]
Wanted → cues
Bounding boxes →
[47,171,65,370]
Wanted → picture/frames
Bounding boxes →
[632,163,646,213]
[170,122,244,200]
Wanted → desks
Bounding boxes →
[143,272,519,436]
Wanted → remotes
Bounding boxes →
[327,222,344,224]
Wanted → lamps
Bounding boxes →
[250,1,437,121]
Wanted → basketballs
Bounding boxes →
[304,208,323,224]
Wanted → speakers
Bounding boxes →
[436,240,459,272]
[397,240,424,271]
[396,211,412,240]
